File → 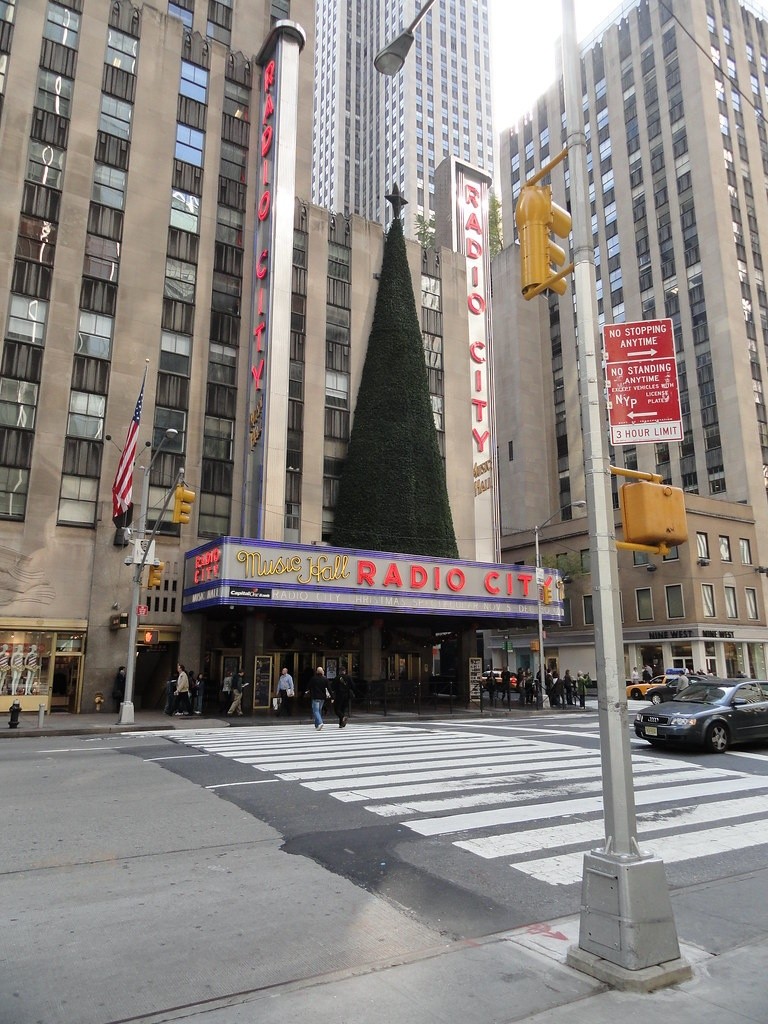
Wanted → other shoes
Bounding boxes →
[316,723,323,730]
[195,711,201,713]
[226,713,234,717]
[238,714,246,717]
[174,712,183,715]
[339,717,347,728]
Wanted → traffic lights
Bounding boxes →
[144,630,160,645]
[148,561,165,587]
[173,484,196,525]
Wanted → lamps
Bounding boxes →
[563,574,573,584]
[695,558,712,566]
[755,566,768,576]
[646,563,658,572]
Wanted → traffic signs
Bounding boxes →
[605,358,685,446]
[602,319,675,364]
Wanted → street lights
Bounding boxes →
[118,428,179,720]
[535,500,585,706]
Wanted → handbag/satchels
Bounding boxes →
[112,690,124,699]
[348,689,355,698]
[286,689,295,698]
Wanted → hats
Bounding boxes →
[577,671,582,677]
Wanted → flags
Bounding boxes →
[111,379,143,520]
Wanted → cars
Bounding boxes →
[647,675,721,705]
[634,676,768,754]
[481,669,518,693]
[626,668,697,701]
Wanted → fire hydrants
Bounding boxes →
[95,691,105,713]
[8,699,22,729]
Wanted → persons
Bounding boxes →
[632,667,640,684]
[0,643,11,695]
[736,670,745,678]
[690,669,694,675]
[24,645,39,696]
[299,663,334,731]
[11,644,25,695]
[676,670,689,695]
[113,666,126,712]
[329,666,355,728]
[500,665,511,706]
[642,666,652,681]
[513,664,590,709]
[275,668,294,716]
[163,664,204,716]
[697,668,714,676]
[220,669,245,716]
[486,671,496,706]
